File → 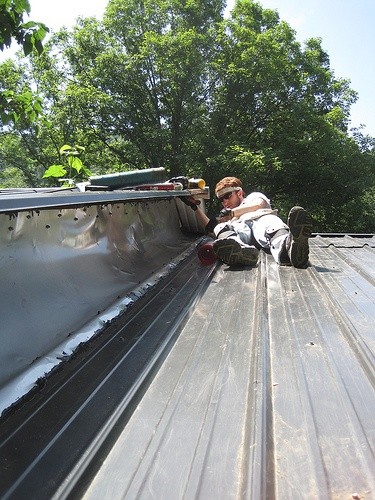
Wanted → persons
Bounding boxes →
[176,176,313,269]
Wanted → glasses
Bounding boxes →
[218,192,235,201]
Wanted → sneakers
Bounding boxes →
[214,237,258,266]
[286,206,311,267]
